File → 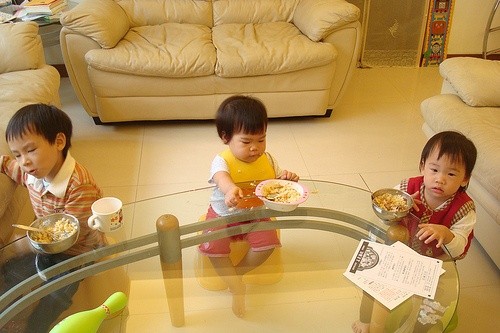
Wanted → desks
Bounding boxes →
[0,0,79,28]
[0,179,459,333]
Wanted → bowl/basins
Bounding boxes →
[26,213,80,254]
[255,179,309,212]
[371,188,414,225]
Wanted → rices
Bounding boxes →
[373,192,409,212]
[264,181,302,203]
[29,218,76,243]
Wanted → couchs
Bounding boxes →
[0,22,60,258]
[420,56,500,271]
[59,0,362,126]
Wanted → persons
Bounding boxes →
[351,130,478,333]
[0,102,109,333]
[199,95,299,319]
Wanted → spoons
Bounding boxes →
[239,193,279,200]
[12,224,69,241]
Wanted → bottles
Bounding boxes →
[48,292,127,333]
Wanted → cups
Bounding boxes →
[88,197,123,232]
